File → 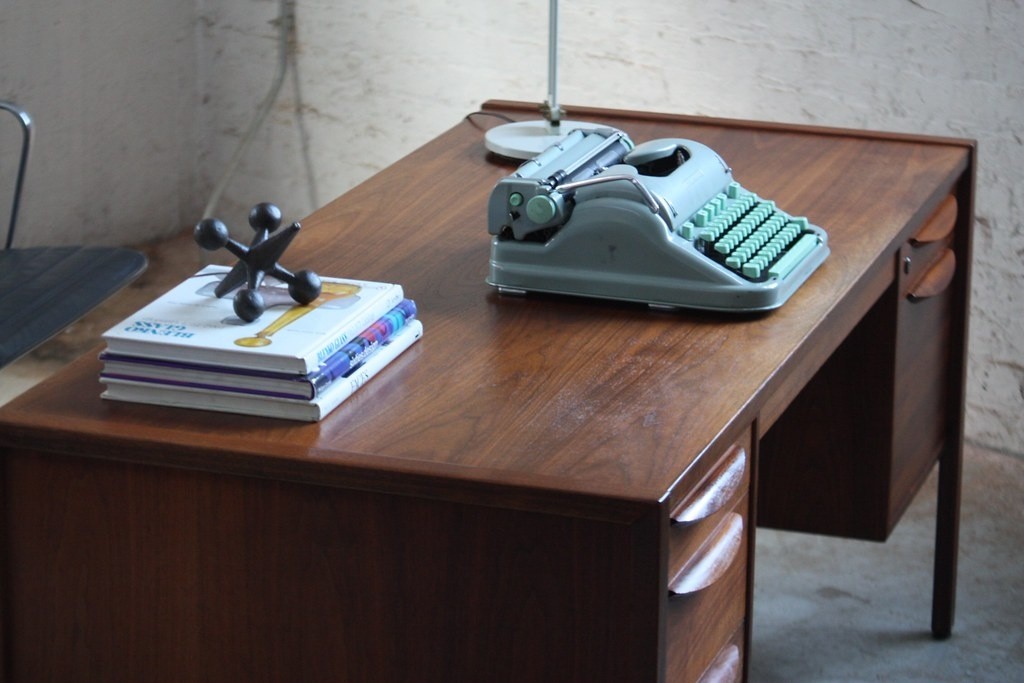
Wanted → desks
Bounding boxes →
[0,99,980,683]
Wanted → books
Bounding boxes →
[98,263,423,423]
